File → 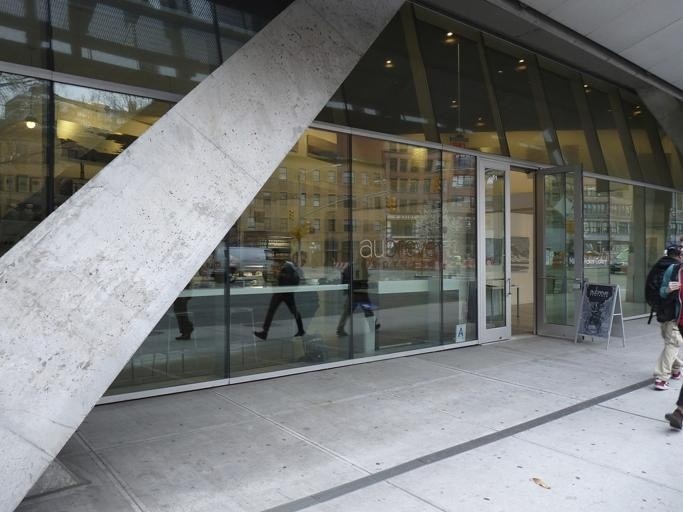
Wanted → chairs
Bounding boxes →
[665,409,683,429]
[293,330,304,337]
[253,331,266,340]
[336,330,347,335]
[368,324,380,332]
[175,332,190,340]
[654,379,670,391]
[672,371,681,379]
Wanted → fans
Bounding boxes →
[575,281,619,339]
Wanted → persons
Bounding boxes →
[645,244,683,390]
[253,255,306,341]
[336,252,381,336]
[174,283,194,340]
[659,237,683,429]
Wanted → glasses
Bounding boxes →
[23,86,39,130]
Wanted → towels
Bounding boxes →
[352,312,376,353]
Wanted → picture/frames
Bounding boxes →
[485,284,520,320]
[353,278,468,341]
[177,284,349,296]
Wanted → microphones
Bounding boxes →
[151,312,197,376]
[230,307,258,368]
